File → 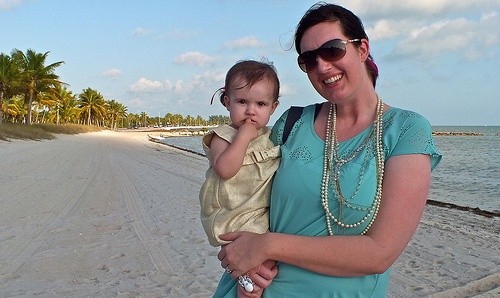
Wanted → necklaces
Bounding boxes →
[320,94,385,236]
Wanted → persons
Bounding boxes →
[198,60,282,298]
[209,0,443,298]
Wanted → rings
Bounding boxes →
[225,266,232,274]
[237,276,255,292]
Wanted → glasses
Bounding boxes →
[298,38,359,73]
